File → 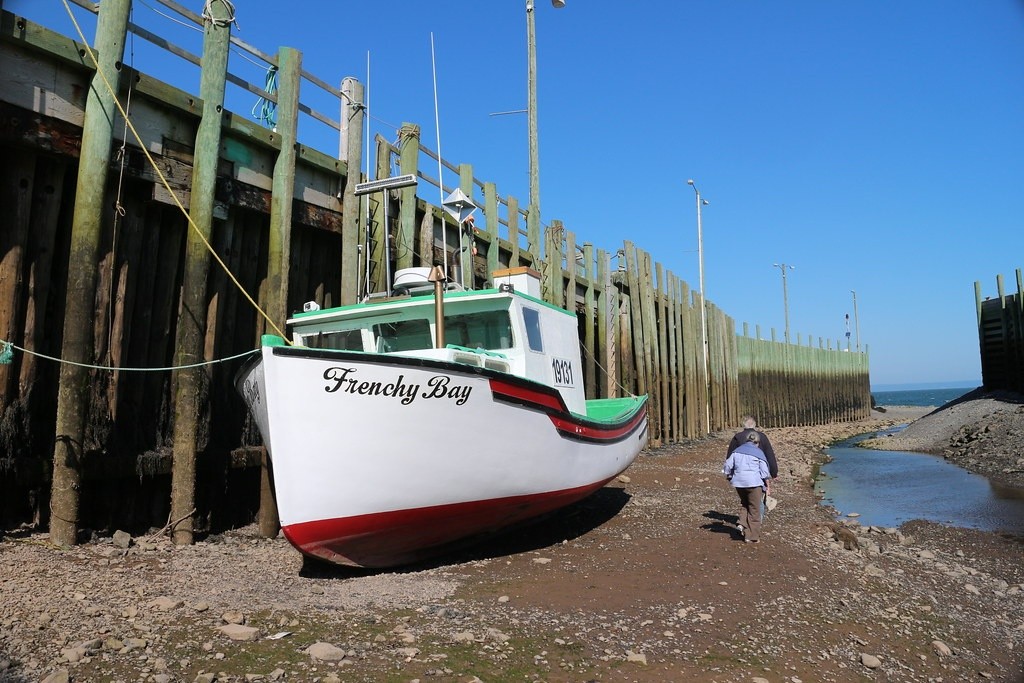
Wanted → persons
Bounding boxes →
[725,432,771,544]
[726,415,778,526]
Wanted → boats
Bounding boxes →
[230,29,650,570]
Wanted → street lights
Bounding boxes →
[850,289,860,349]
[526,0,565,267]
[687,179,712,436]
[774,262,795,334]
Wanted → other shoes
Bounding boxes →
[734,525,744,539]
[744,539,760,543]
